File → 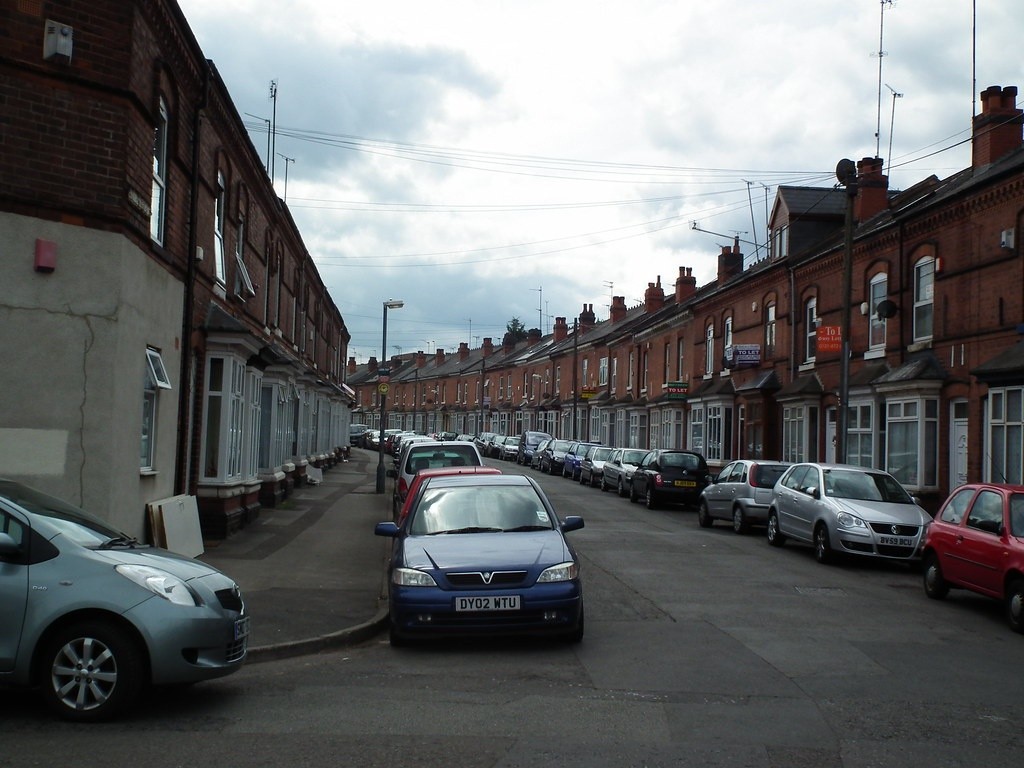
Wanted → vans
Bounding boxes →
[385,440,483,523]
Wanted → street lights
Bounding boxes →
[376,299,405,484]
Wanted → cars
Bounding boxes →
[580,446,617,487]
[398,467,502,536]
[629,448,710,513]
[921,482,1024,631]
[562,441,601,479]
[1,478,249,714]
[499,436,522,461]
[349,422,474,467]
[376,475,586,651]
[529,439,551,470]
[488,434,508,457]
[600,448,650,496]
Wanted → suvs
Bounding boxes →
[517,429,553,466]
[767,461,934,571]
[697,458,797,534]
[476,432,497,456]
[541,437,579,475]
